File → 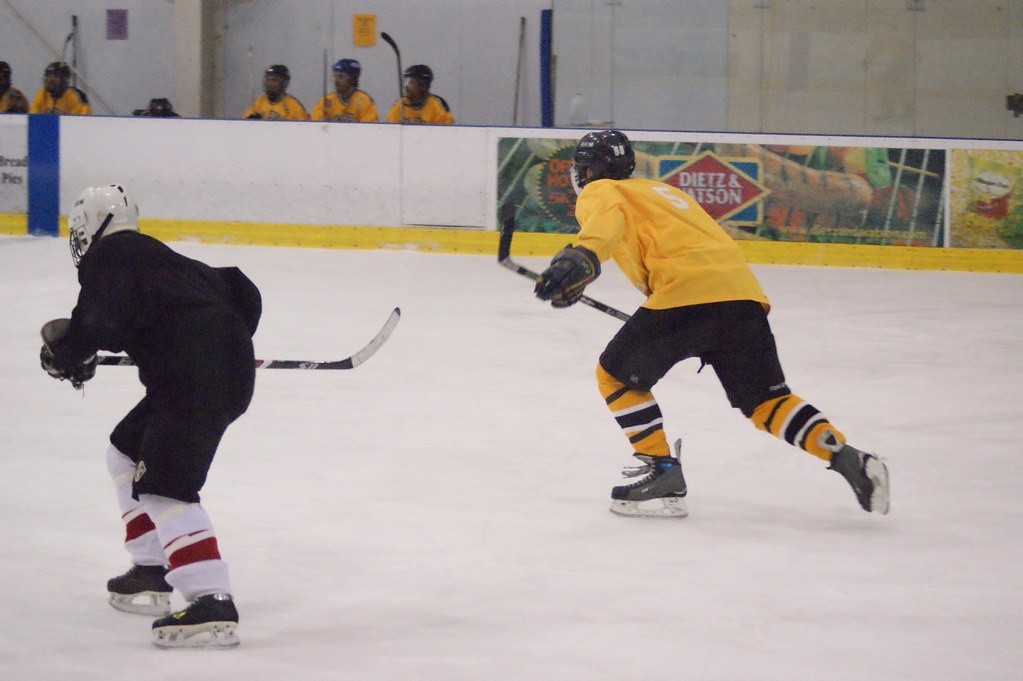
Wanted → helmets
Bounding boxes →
[333,59,360,88]
[68,184,139,269]
[403,65,431,92]
[570,130,635,196]
[265,65,289,90]
[45,62,71,86]
[151,99,171,117]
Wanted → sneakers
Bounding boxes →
[609,438,689,519]
[151,594,239,649]
[107,565,173,616]
[819,432,889,514]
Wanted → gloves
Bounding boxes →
[39,318,98,389]
[534,243,600,308]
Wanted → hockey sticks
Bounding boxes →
[48,14,80,110]
[495,198,631,323]
[249,44,257,117]
[322,49,328,122]
[382,32,403,120]
[44,306,402,372]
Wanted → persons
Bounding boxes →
[388,64,454,125]
[0,60,29,113]
[41,182,262,653]
[243,64,309,119]
[311,59,379,122]
[533,131,890,521]
[29,62,92,116]
